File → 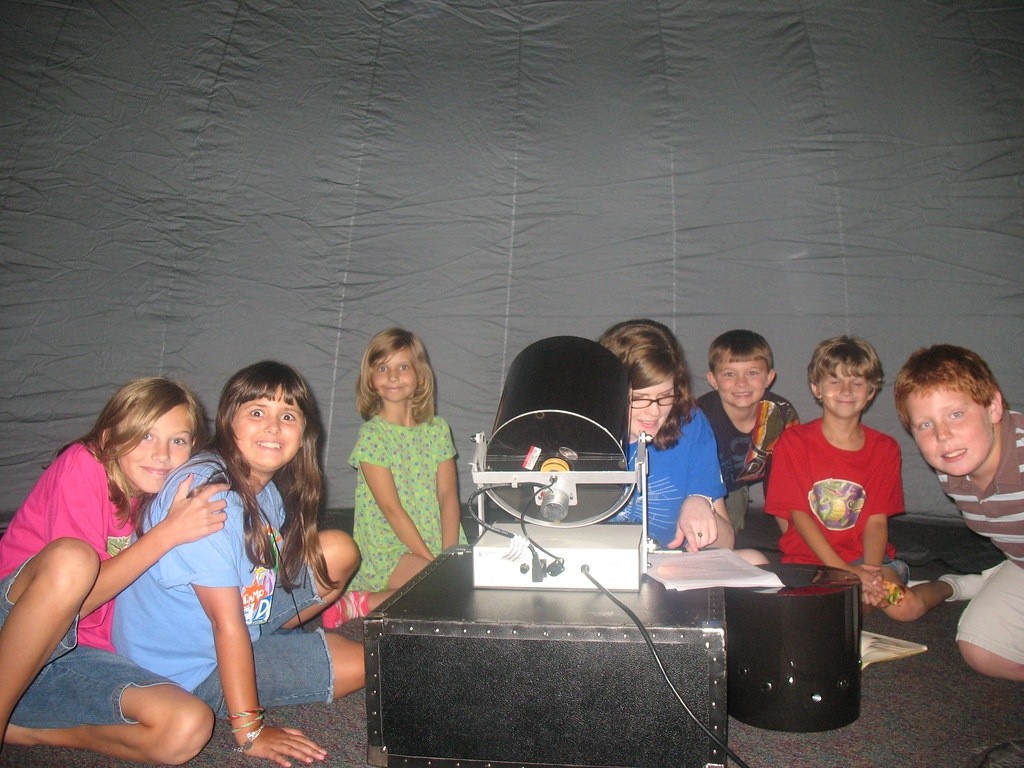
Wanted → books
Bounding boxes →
[859,629,928,670]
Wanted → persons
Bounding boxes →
[766,336,990,623]
[695,329,803,535]
[319,327,469,628]
[596,319,736,551]
[894,342,1023,682]
[109,360,366,766]
[0,377,233,768]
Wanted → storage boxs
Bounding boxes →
[364,544,728,768]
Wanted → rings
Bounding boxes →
[695,532,702,537]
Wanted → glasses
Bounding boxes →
[630,390,682,409]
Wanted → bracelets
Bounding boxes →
[227,706,266,754]
[689,494,715,514]
